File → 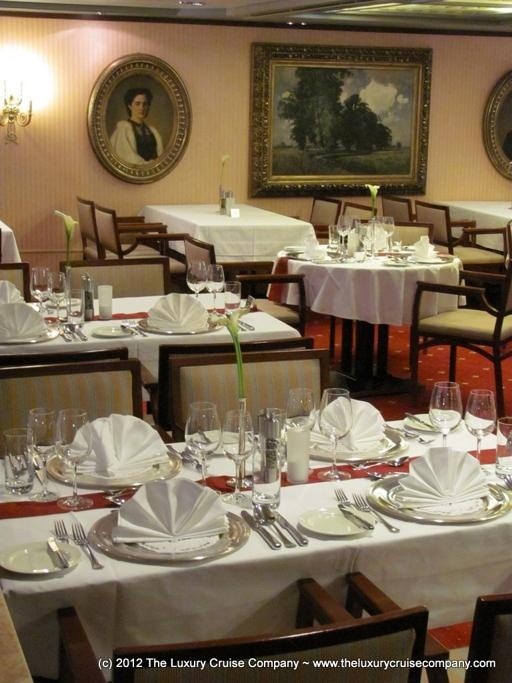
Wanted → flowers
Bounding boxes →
[218,155,233,200]
[365,184,379,221]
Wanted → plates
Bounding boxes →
[139,316,227,334]
[283,244,331,264]
[0,330,60,344]
[299,506,369,538]
[403,245,454,264]
[404,414,436,431]
[1,542,81,574]
[369,471,512,522]
[93,326,134,336]
[87,509,249,565]
[45,445,184,489]
[313,416,409,462]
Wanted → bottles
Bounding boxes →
[81,272,94,321]
[220,191,236,216]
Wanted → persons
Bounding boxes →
[110,87,163,165]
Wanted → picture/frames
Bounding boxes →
[86,53,193,185]
[246,42,432,198]
[482,69,512,179]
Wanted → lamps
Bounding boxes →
[0,69,33,145]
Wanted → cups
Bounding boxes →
[69,289,86,326]
[3,425,34,495]
[98,284,114,318]
[225,280,243,314]
[496,415,512,479]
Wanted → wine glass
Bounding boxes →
[326,210,411,266]
[429,380,498,479]
[185,385,353,510]
[29,264,67,333]
[185,258,225,321]
[29,403,94,508]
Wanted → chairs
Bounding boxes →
[3,196,512,683]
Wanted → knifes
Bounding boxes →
[404,411,434,429]
[57,322,88,342]
[242,502,308,550]
[47,536,69,568]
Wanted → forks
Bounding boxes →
[70,521,103,569]
[336,490,403,537]
[54,519,70,543]
[383,423,434,445]
